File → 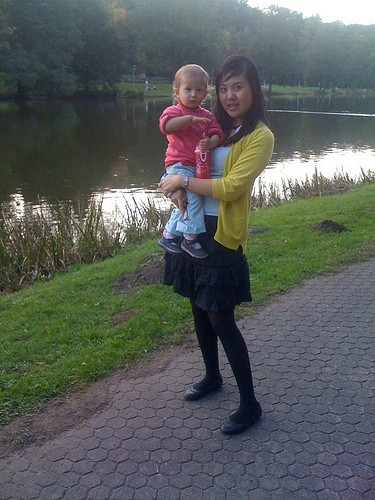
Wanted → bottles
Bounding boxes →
[194,142,210,179]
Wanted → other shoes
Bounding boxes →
[158,236,182,255]
[221,402,262,434]
[181,238,209,260]
[184,374,223,400]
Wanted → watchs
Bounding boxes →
[184,176,189,190]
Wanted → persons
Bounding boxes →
[159,55,276,434]
[143,78,157,91]
[159,63,224,259]
